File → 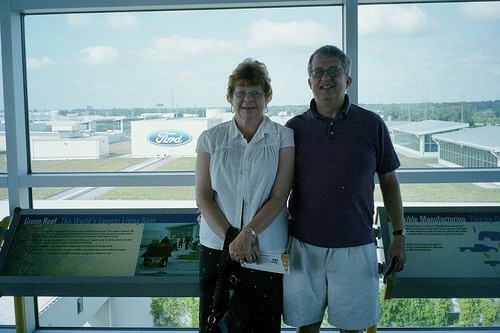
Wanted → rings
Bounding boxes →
[402,264,406,267]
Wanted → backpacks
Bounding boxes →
[205,226,265,333]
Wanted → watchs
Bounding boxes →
[392,228,407,238]
[243,225,257,238]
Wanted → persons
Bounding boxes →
[158,233,193,250]
[197,45,408,332]
[196,58,295,333]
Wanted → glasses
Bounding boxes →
[310,66,349,78]
[233,90,265,97]
